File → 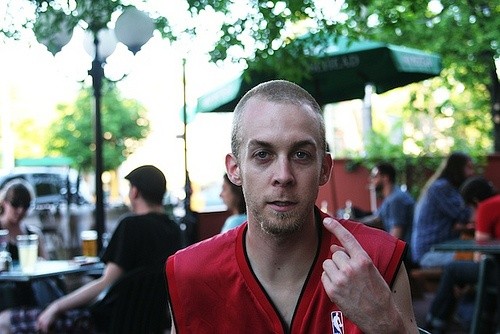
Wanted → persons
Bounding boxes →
[164,80,419,334]
[358,152,500,334]
[219,173,248,232]
[0,184,49,311]
[0,165,186,334]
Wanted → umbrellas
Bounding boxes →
[12,152,72,168]
[195,30,441,113]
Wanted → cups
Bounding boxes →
[81,231,97,257]
[16,235,39,271]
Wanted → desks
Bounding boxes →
[432,241,500,334]
[0,260,104,310]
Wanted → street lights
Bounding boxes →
[32,0,155,260]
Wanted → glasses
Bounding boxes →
[9,200,31,211]
